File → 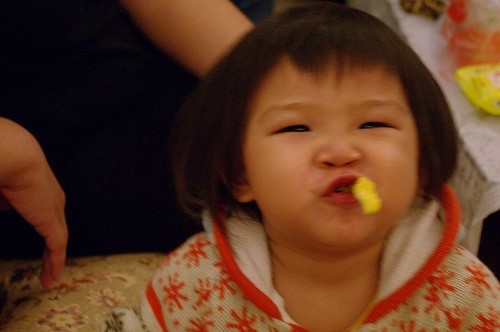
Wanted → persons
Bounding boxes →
[0,113,69,288]
[1,1,277,260]
[135,1,500,332]
